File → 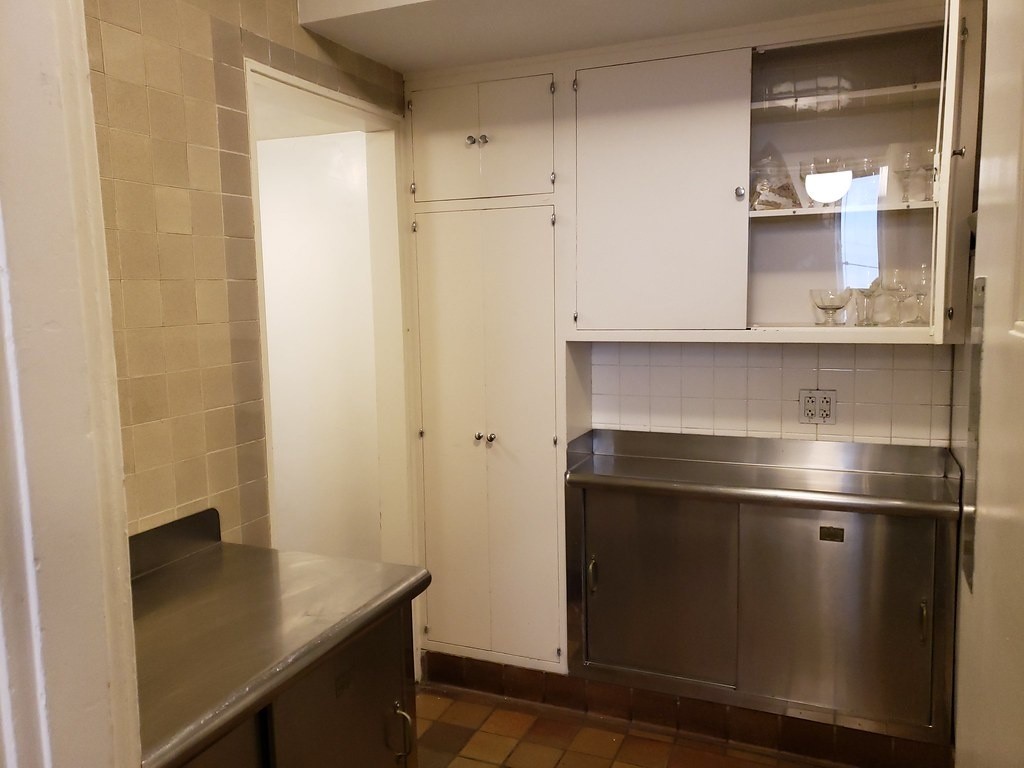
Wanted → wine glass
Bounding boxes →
[809,265,928,326]
[800,150,934,208]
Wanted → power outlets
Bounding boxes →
[799,389,837,425]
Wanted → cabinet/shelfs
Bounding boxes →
[130,532,434,768]
[406,52,592,672]
[565,0,985,345]
[566,427,965,768]
